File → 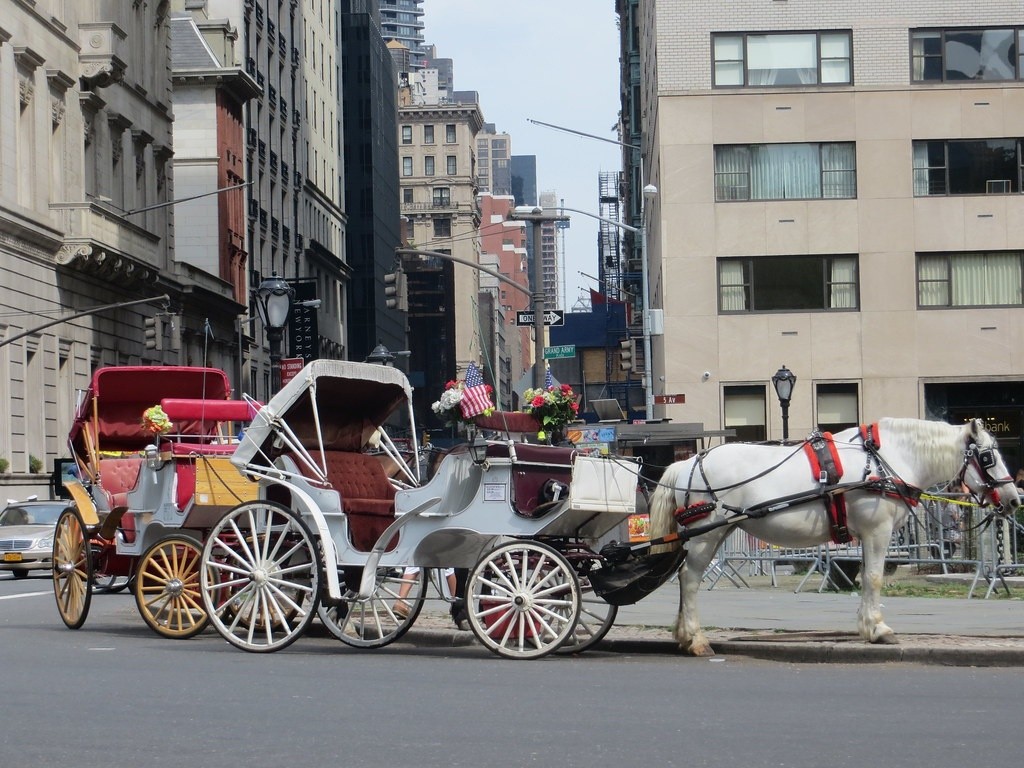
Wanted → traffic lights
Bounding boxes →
[619,339,636,374]
[385,272,405,315]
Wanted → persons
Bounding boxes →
[928,467,1024,559]
[388,446,456,618]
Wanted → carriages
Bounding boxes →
[198,357,1022,661]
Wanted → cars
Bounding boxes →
[0,496,77,581]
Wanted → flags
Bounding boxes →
[461,363,494,419]
[545,368,552,389]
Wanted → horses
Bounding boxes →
[648,417,1020,658]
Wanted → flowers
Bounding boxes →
[523,384,580,442]
[431,379,496,442]
[141,404,173,437]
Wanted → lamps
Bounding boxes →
[465,429,489,469]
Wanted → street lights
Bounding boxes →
[251,267,297,473]
[771,363,797,440]
[513,184,658,423]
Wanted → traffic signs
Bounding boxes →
[515,309,564,328]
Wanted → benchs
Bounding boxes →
[284,449,398,516]
[160,399,264,461]
[86,458,145,516]
[475,411,588,468]
